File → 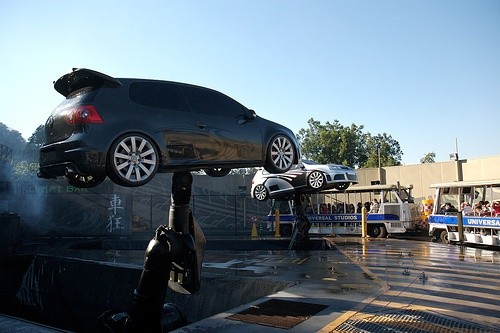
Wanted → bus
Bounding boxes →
[265,182,415,238]
[429,180,500,250]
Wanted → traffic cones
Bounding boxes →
[251,223,259,237]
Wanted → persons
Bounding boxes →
[285,199,389,215]
[440,200,500,218]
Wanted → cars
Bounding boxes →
[36,69,301,187]
[250,160,360,201]
